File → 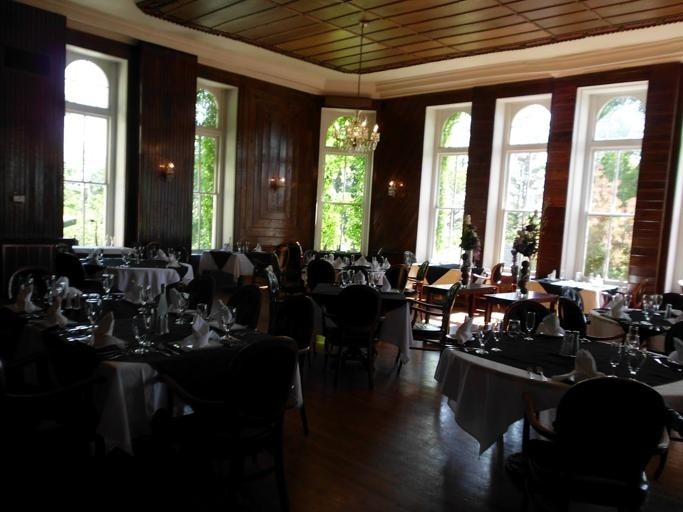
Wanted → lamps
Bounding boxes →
[270,176,287,194]
[387,179,408,199]
[330,11,387,153]
[160,160,179,186]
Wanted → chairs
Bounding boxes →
[502,376,672,511]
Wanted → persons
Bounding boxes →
[667,408,683,435]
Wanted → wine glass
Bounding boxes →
[26,274,240,354]
[87,238,192,270]
[287,269,385,292]
[458,310,646,382]
[601,287,681,329]
[305,253,392,263]
[218,235,252,255]
[546,268,608,290]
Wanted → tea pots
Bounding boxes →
[557,327,589,359]
